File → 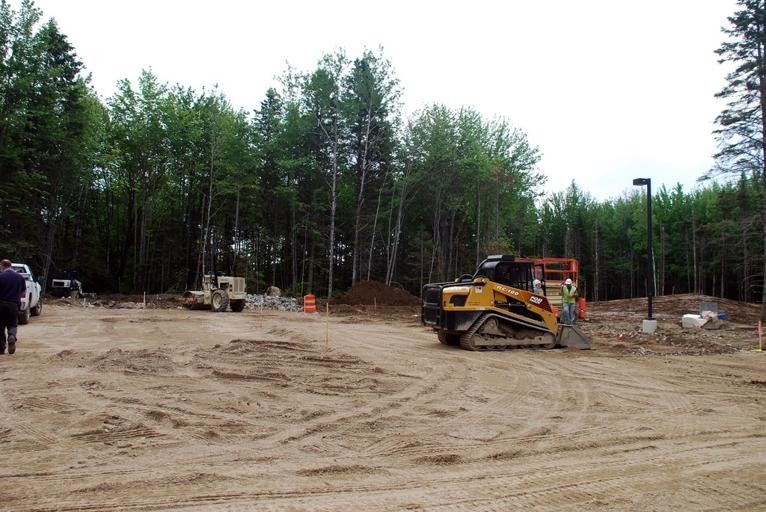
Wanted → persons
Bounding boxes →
[0,258,27,354]
[560,278,578,325]
[533,279,544,294]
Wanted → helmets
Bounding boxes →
[533,279,541,287]
[566,278,572,284]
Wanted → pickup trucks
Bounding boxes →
[10,262,42,324]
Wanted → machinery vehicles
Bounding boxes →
[420,255,592,350]
[181,274,246,311]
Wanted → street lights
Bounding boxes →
[632,177,653,321]
